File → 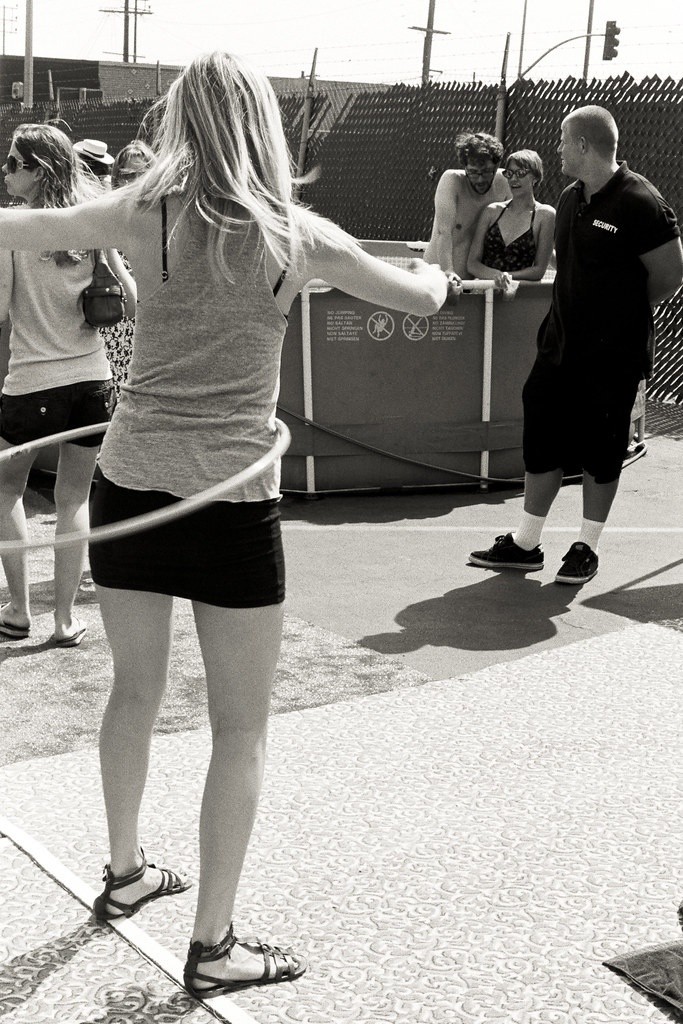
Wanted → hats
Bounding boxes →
[72,138,116,164]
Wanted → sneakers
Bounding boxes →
[555,541,599,584]
[468,531,545,571]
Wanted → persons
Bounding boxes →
[0,46,450,999]
[464,147,557,290]
[467,105,683,584]
[0,122,160,645]
[422,130,513,296]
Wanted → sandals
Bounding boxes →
[93,847,194,920]
[182,921,309,1000]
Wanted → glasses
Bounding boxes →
[118,169,146,181]
[6,155,32,174]
[465,163,497,175]
[502,167,532,179]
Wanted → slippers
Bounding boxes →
[0,603,31,637]
[56,615,88,646]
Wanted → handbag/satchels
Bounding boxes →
[82,246,127,328]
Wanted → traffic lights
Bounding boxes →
[607,26,620,58]
